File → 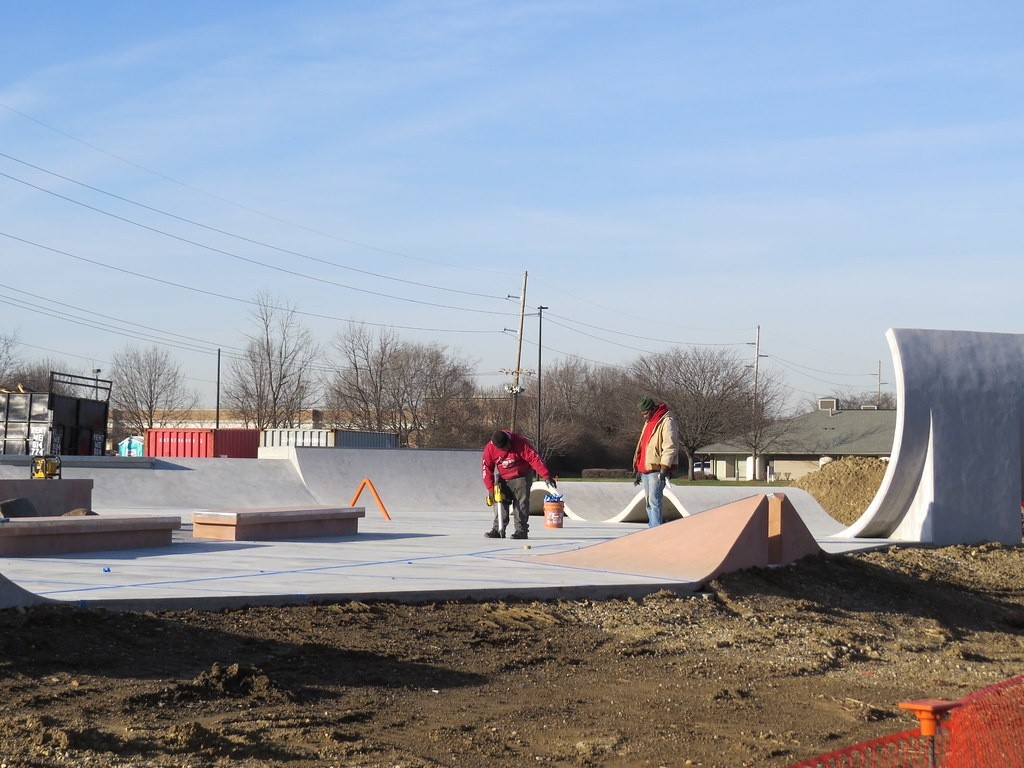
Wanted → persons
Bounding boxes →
[633,397,678,527]
[482,429,558,540]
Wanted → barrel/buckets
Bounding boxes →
[544,501,564,528]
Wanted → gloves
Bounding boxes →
[489,493,494,501]
[546,478,557,488]
[633,473,641,486]
[660,470,670,480]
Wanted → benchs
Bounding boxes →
[192,503,366,541]
[0,512,182,558]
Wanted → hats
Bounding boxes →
[637,396,654,411]
[492,430,508,449]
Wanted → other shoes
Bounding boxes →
[484,529,505,538]
[510,531,528,538]
